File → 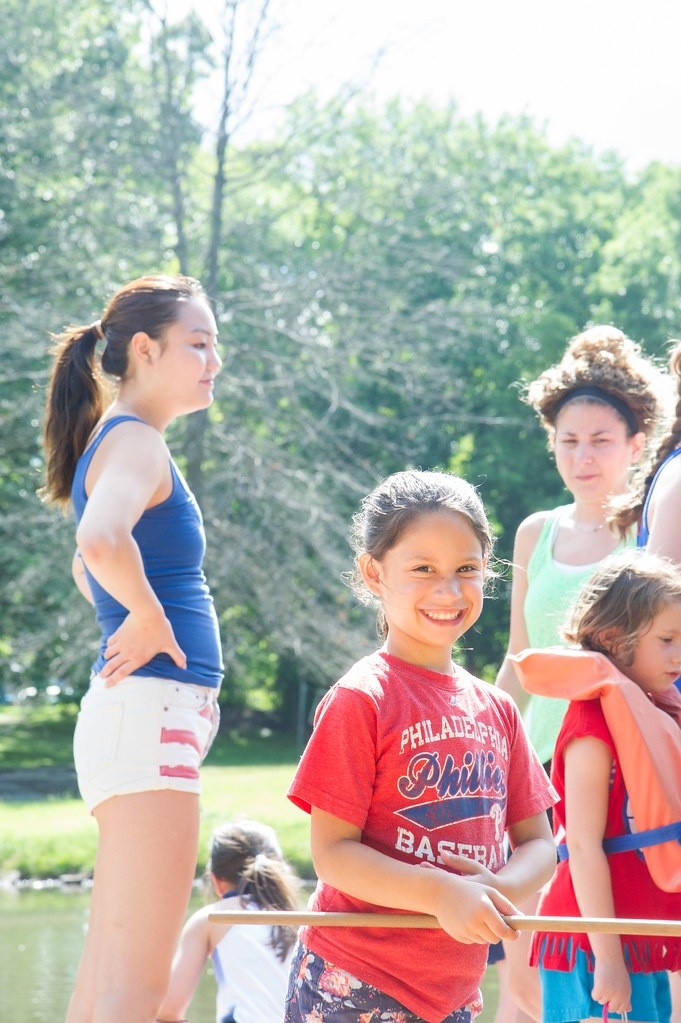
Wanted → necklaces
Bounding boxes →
[568,503,606,534]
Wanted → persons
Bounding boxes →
[157,820,304,1022]
[38,273,224,1022]
[531,548,680,1020]
[495,322,677,1021]
[286,469,561,1022]
[605,335,680,566]
